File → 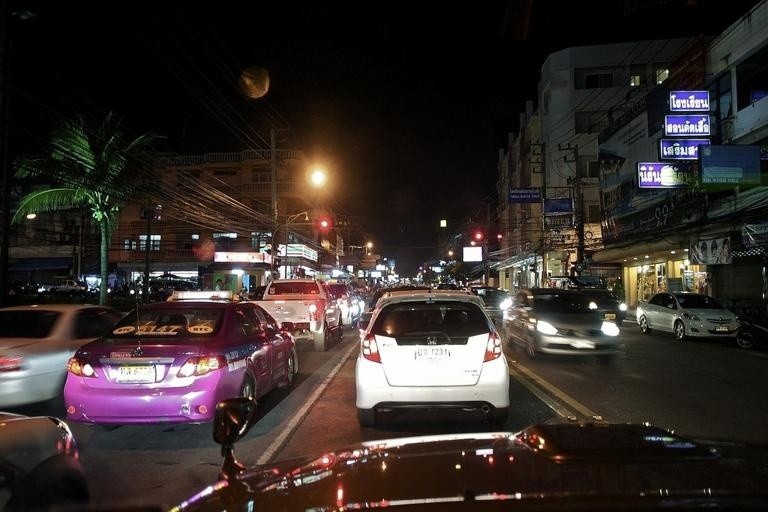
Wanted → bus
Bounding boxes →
[137,277,196,302]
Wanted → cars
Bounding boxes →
[638,292,768,349]
[64,291,299,443]
[0,306,124,407]
[327,282,511,425]
[501,288,626,357]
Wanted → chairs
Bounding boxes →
[167,314,188,333]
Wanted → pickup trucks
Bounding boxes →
[42,281,79,292]
[244,279,344,350]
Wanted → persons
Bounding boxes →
[215,277,225,290]
[120,281,134,298]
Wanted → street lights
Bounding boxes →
[285,212,309,280]
